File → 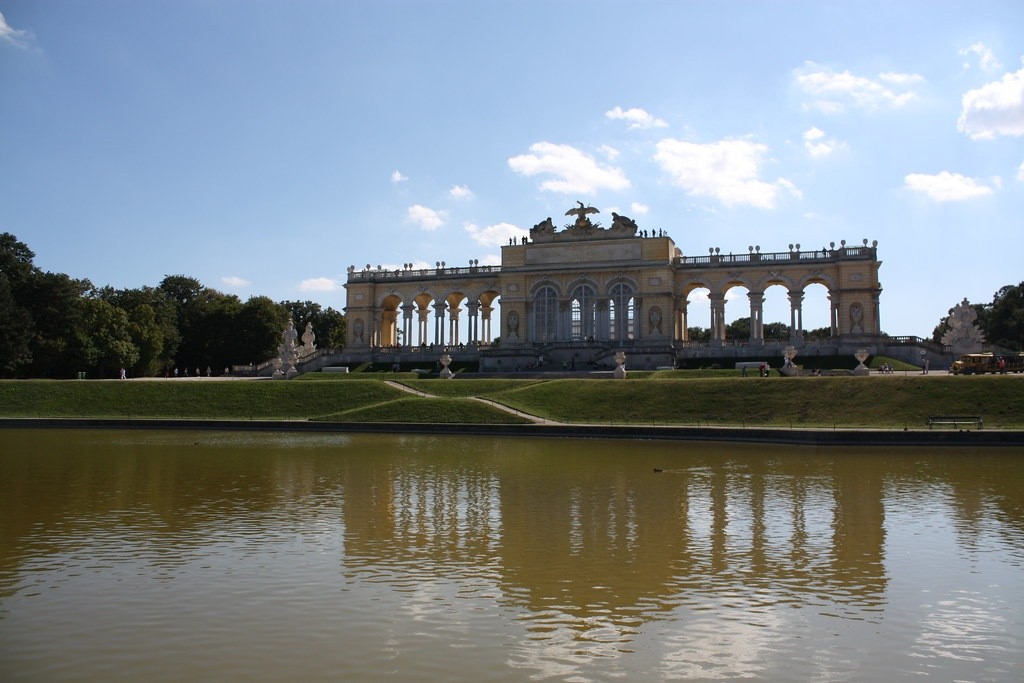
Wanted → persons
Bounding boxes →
[991,356,997,374]
[369,364,373,373]
[515,360,543,371]
[563,357,576,371]
[165,367,229,378]
[922,357,929,374]
[1000,357,1005,373]
[382,342,468,351]
[880,362,893,374]
[393,363,399,372]
[587,356,612,370]
[121,368,126,379]
[787,358,792,368]
[759,362,770,376]
[742,366,747,377]
[811,368,821,375]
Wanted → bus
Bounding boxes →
[950,352,1024,375]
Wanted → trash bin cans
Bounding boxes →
[82,372,86,379]
[76,371,81,379]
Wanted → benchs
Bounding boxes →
[735,361,767,368]
[929,416,983,430]
[322,366,350,373]
[878,369,892,373]
[657,366,674,370]
[590,371,614,379]
[411,369,432,374]
